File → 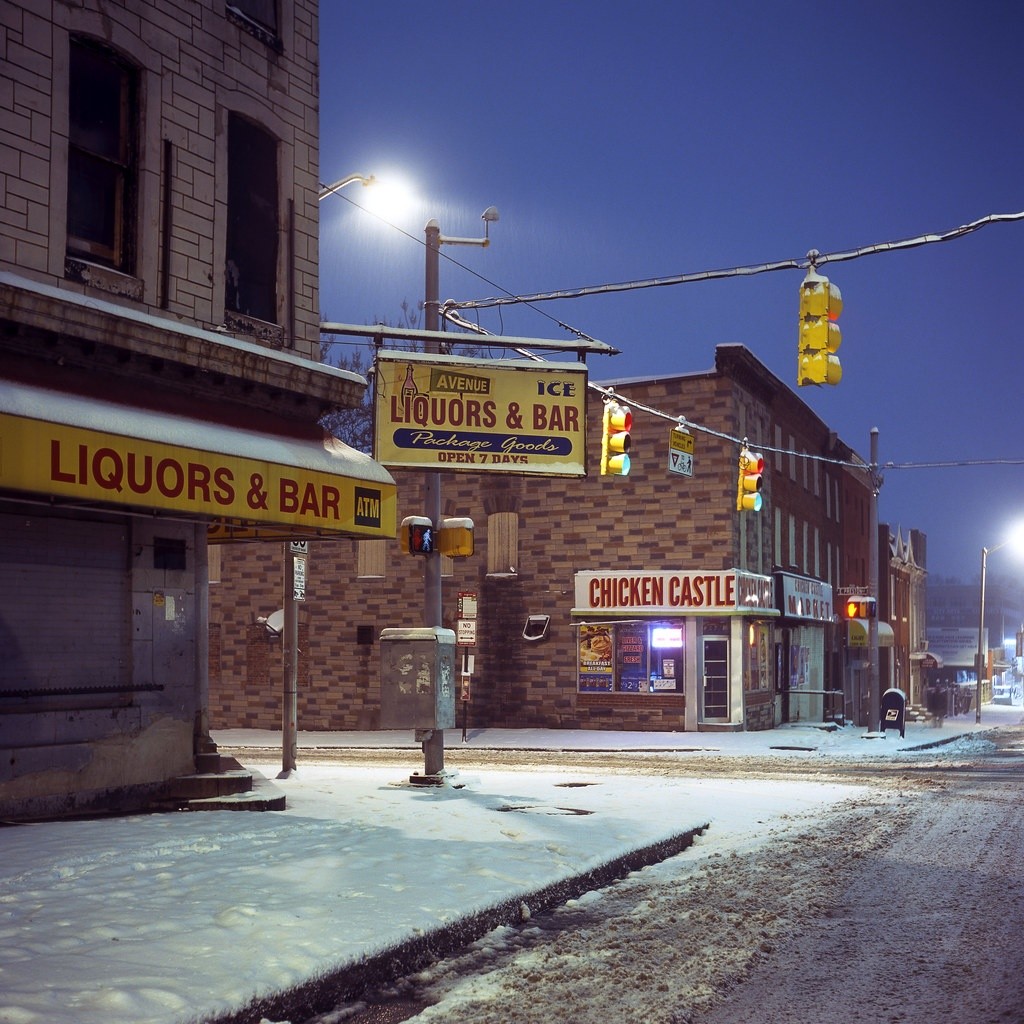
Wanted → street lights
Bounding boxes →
[974,532,1023,721]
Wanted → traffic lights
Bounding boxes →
[600,403,633,475]
[798,276,843,383]
[861,598,877,617]
[400,515,434,557]
[847,598,860,619]
[737,448,764,514]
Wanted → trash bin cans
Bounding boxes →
[881,688,905,739]
